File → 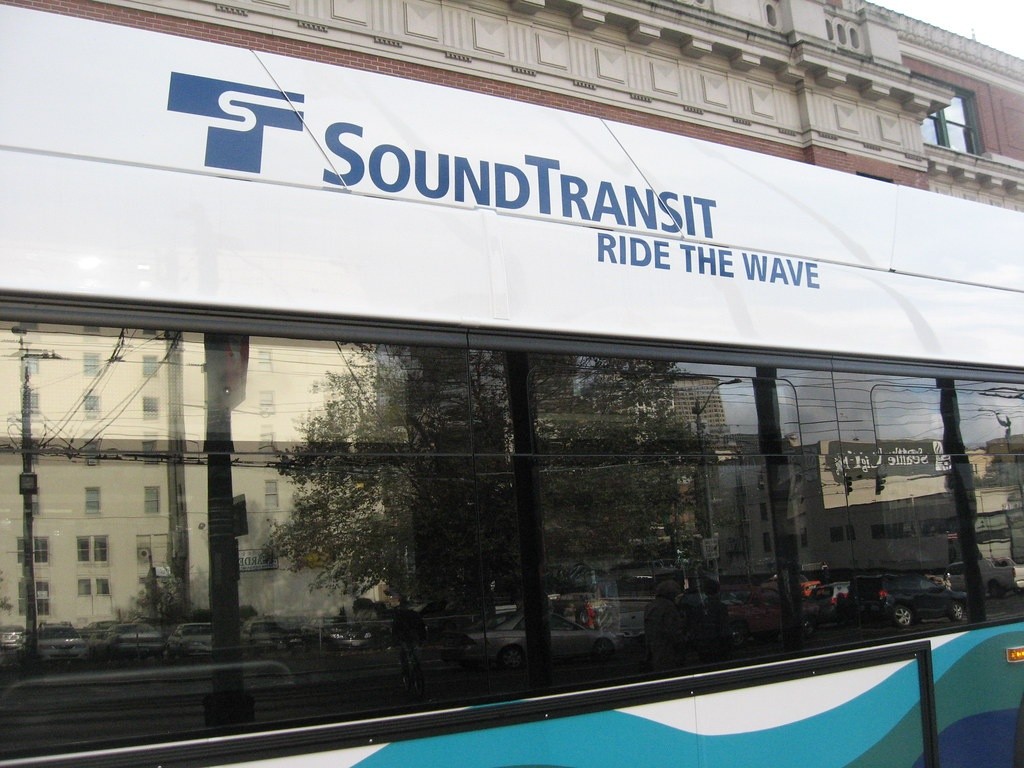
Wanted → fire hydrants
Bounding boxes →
[583,602,595,628]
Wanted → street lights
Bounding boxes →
[695,378,744,578]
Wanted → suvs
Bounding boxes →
[845,574,969,630]
[36,620,89,664]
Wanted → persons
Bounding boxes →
[394,594,430,709]
[578,598,598,630]
[774,557,807,651]
[936,569,956,592]
[641,569,728,675]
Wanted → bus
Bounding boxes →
[0,5,1023,766]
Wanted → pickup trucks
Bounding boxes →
[944,557,1024,599]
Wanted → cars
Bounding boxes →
[441,610,619,674]
[807,582,850,624]
[103,623,164,661]
[239,618,288,648]
[719,584,820,648]
[0,625,26,650]
[82,620,120,638]
[165,622,213,658]
[334,622,393,652]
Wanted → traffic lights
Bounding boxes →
[845,476,853,494]
[876,473,886,495]
